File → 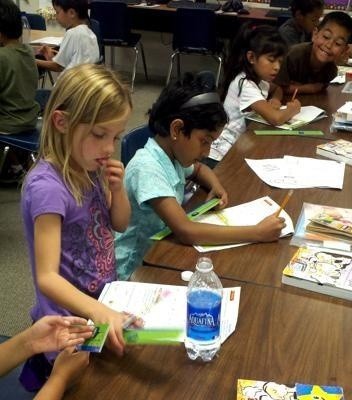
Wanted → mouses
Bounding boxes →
[237,9,249,15]
[147,2,153,5]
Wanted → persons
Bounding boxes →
[31,0,102,119]
[1,313,94,400]
[19,63,145,375]
[106,75,285,284]
[203,24,302,174]
[0,0,40,191]
[270,12,351,99]
[275,0,351,66]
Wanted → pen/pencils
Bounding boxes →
[291,88,298,102]
[277,190,293,217]
[121,309,145,329]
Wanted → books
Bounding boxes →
[330,100,352,133]
[316,138,352,166]
[281,206,352,307]
[235,378,345,400]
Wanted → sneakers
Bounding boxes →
[1,167,28,186]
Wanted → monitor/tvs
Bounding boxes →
[270,0,289,8]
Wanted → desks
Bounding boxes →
[251,81,352,134]
[144,129,352,295]
[110,0,284,89]
[61,266,352,400]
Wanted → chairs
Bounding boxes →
[0,126,43,176]
[166,9,224,93]
[118,123,154,163]
[88,23,107,68]
[23,13,45,29]
[90,0,154,96]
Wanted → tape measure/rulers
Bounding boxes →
[253,130,325,136]
[122,329,183,345]
[149,198,223,240]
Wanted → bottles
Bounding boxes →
[185,257,224,363]
[20,11,32,53]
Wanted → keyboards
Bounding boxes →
[168,1,221,11]
[265,10,288,17]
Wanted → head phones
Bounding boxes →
[222,0,243,12]
